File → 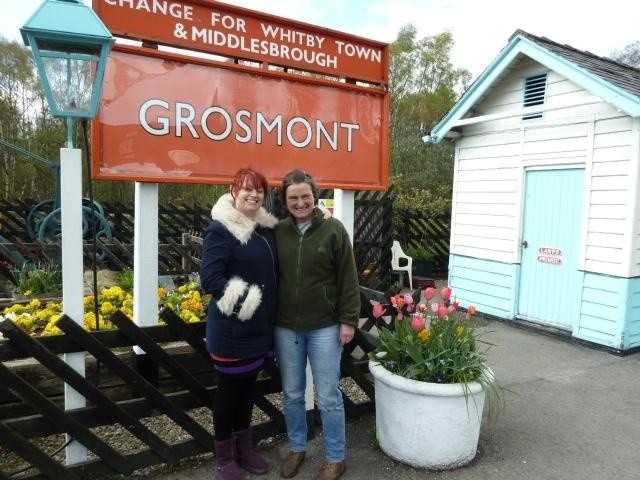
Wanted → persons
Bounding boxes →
[273,168,360,480]
[201,166,279,480]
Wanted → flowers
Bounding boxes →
[4,283,207,337]
[371,283,518,420]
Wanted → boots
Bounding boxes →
[214,435,244,480]
[233,428,269,474]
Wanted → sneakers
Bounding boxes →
[282,451,305,478]
[315,462,346,479]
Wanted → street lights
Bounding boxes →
[16,0,121,467]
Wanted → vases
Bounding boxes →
[367,352,494,471]
